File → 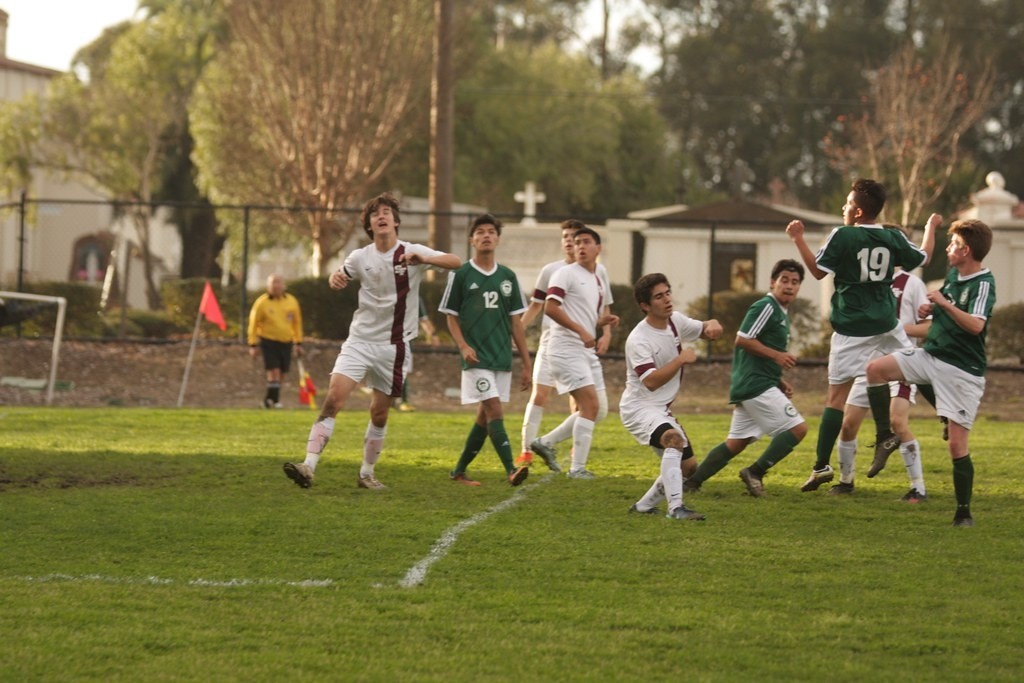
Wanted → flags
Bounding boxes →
[299,368,317,410]
[199,283,226,332]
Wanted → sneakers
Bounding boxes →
[514,452,533,468]
[738,467,765,499]
[800,464,834,492]
[951,516,973,528]
[448,470,481,486]
[824,479,854,496]
[665,504,706,521]
[682,482,703,494]
[566,467,601,481]
[893,488,927,504]
[940,416,949,440]
[529,436,563,473]
[627,502,668,516]
[283,462,316,489]
[356,469,387,491]
[508,466,529,486]
[866,433,901,478]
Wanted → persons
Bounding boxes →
[438,212,531,486]
[247,273,304,409]
[823,219,997,529]
[283,192,461,490]
[786,177,943,493]
[511,219,619,481]
[682,259,808,498]
[620,272,723,521]
